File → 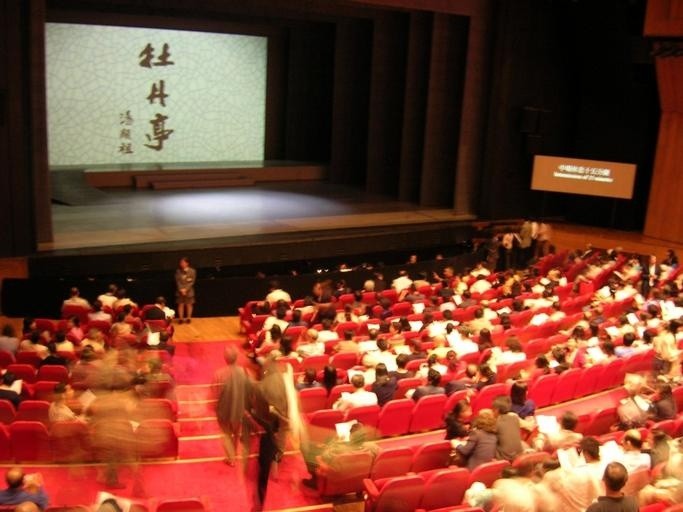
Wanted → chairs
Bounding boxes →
[0,248,683,512]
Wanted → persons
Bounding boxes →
[175,258,196,324]
[209,346,249,466]
[0,285,176,512]
[464,216,551,257]
[250,245,683,512]
